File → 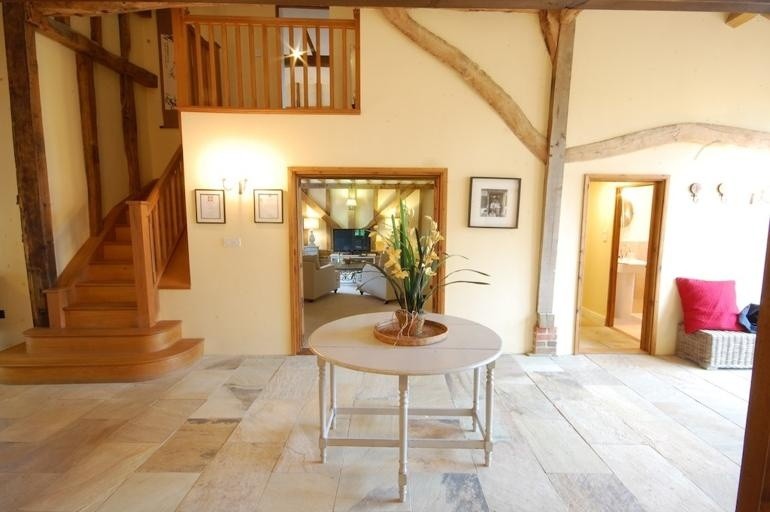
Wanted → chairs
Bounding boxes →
[357,264,430,305]
[303,262,340,303]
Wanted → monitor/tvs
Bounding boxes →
[333,228,371,255]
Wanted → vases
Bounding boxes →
[395,309,425,336]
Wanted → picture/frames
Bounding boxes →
[195,189,226,224]
[468,176,521,229]
[253,189,283,224]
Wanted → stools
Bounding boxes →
[676,322,756,371]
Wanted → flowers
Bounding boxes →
[347,196,491,314]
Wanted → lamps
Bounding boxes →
[345,198,357,210]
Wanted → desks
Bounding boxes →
[308,312,503,502]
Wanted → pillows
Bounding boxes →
[676,277,744,335]
[303,254,320,270]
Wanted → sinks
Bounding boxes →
[617,257,647,273]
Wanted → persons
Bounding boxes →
[481,193,504,216]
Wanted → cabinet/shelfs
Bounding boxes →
[330,254,376,264]
